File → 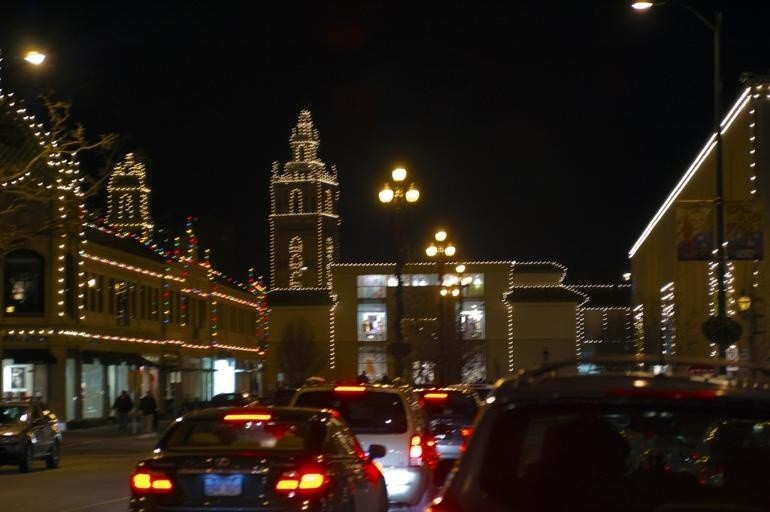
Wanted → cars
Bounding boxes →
[204,391,261,408]
[448,383,496,402]
[413,387,478,459]
[129,407,389,510]
[261,389,292,405]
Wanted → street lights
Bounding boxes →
[449,265,474,361]
[425,229,457,342]
[632,0,726,375]
[378,164,421,380]
[1,50,47,66]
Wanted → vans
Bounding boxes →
[287,383,439,511]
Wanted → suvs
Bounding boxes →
[428,354,770,512]
[0,396,63,473]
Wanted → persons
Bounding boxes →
[111,389,133,433]
[355,369,370,383]
[136,389,157,434]
